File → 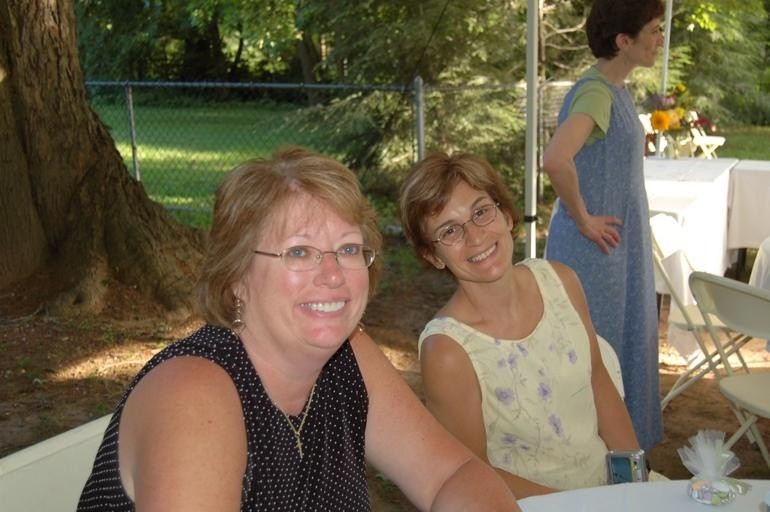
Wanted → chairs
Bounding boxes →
[634,154,741,301]
[2,410,121,510]
[636,109,727,158]
[631,211,751,414]
[586,339,624,402]
[685,272,770,477]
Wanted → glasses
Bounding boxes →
[252,240,378,273]
[422,197,500,247]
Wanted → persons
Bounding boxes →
[397,152,671,499]
[544,0,673,490]
[75,146,524,512]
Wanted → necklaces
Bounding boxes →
[261,370,316,461]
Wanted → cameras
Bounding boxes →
[605,449,648,485]
